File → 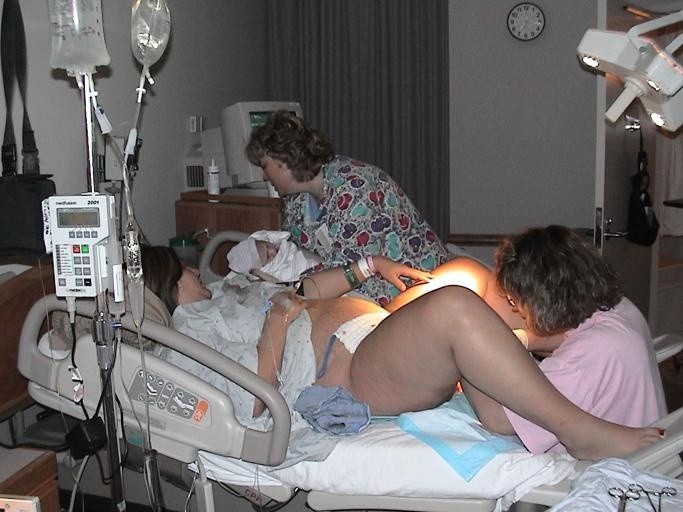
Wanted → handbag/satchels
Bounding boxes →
[626,133,660,248]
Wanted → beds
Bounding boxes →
[17,232,682,509]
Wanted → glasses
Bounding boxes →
[506,296,517,308]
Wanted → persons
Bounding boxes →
[121,242,666,467]
[244,107,450,305]
[224,237,280,278]
[456,223,669,455]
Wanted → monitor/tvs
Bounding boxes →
[192,100,305,200]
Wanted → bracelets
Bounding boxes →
[356,257,374,279]
[340,263,360,291]
[365,253,376,273]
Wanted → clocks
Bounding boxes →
[506,1,546,41]
